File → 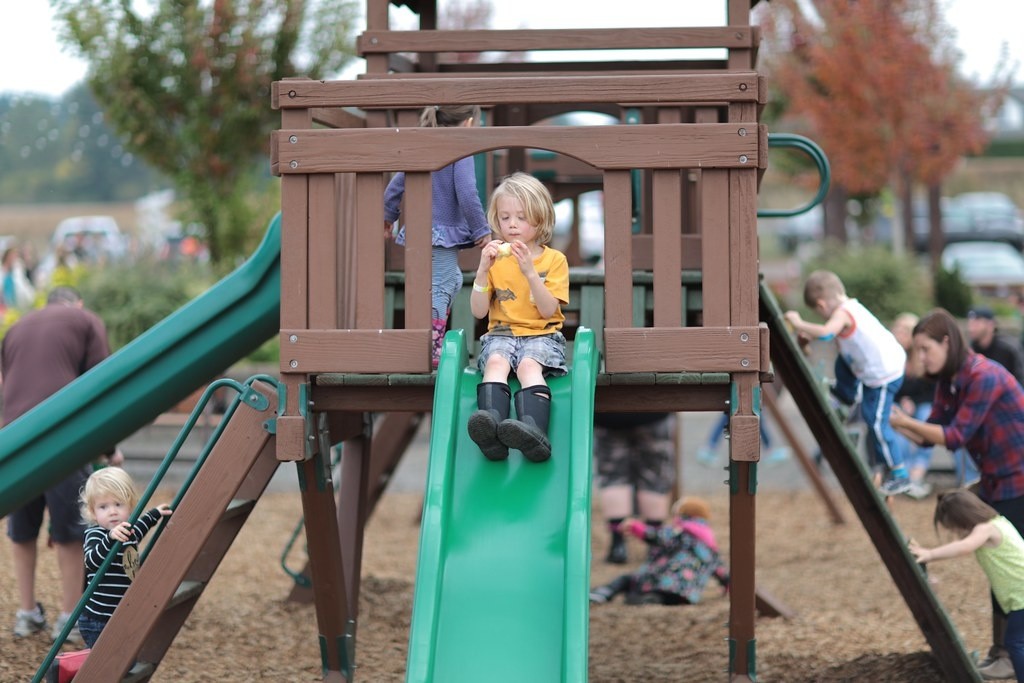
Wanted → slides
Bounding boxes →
[2,207,284,520]
[404,321,601,683]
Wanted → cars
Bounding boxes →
[940,241,1024,309]
[51,217,124,258]
[136,188,208,265]
[782,191,1024,253]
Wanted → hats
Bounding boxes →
[967,308,999,333]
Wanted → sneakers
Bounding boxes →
[12,602,51,637]
[51,614,85,644]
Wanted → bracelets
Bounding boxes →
[473,282,489,292]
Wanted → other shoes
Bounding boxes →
[822,378,854,419]
[879,478,913,495]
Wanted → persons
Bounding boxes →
[468,169,570,461]
[907,489,1024,683]
[698,411,791,469]
[383,105,491,368]
[888,308,1024,679]
[783,267,913,495]
[0,236,44,310]
[77,467,173,673]
[589,496,730,606]
[0,284,113,642]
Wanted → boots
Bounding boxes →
[977,609,1019,680]
[467,382,513,461]
[45,648,92,683]
[647,520,661,557]
[431,318,446,366]
[498,385,552,462]
[589,585,616,604]
[608,516,627,562]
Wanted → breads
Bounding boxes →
[496,242,513,256]
